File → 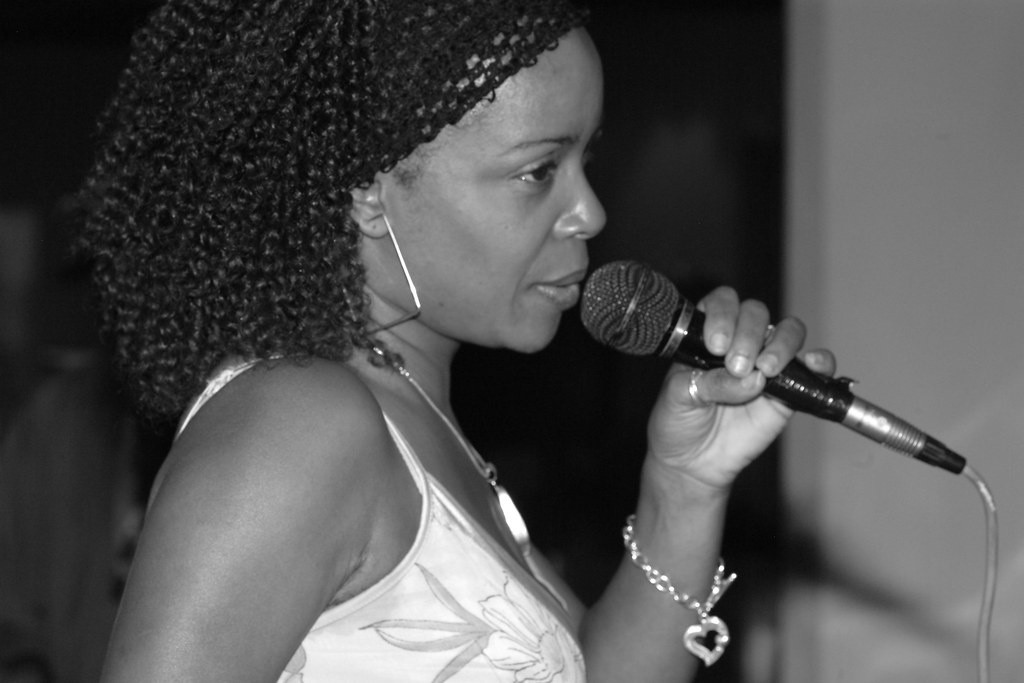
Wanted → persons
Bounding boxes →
[57,0,837,683]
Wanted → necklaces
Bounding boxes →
[376,347,568,611]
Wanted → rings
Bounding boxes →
[688,368,707,408]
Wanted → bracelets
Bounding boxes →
[622,514,738,667]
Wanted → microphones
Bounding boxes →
[580,259,966,475]
